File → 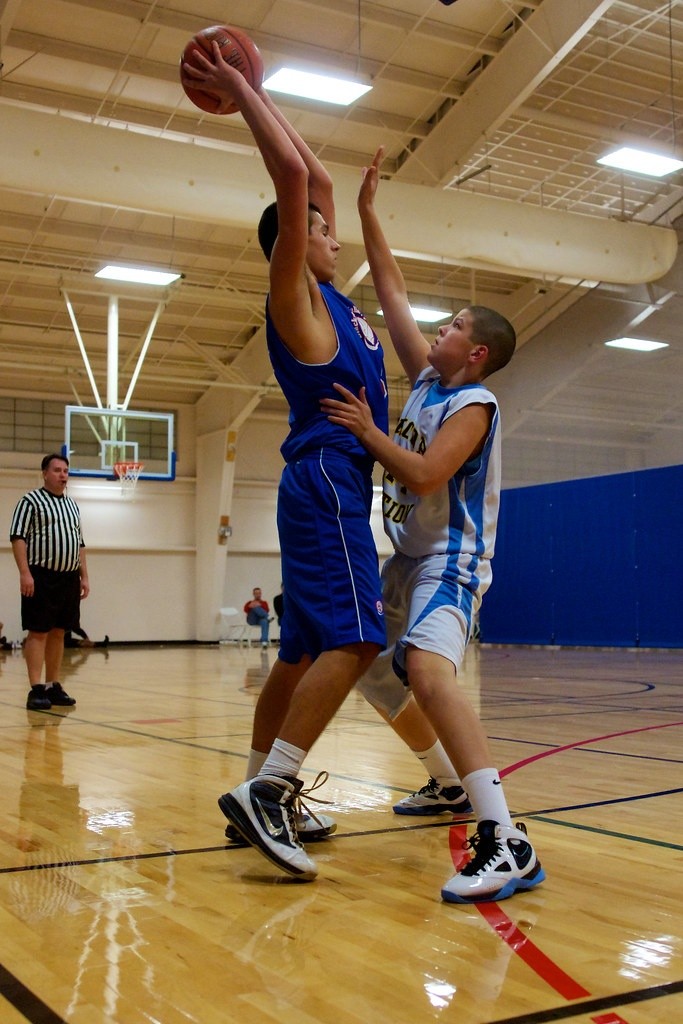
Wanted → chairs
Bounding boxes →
[218,608,272,648]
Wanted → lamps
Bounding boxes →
[595,0,683,177]
[93,205,182,288]
[263,0,374,107]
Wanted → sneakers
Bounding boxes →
[392,777,473,815]
[225,811,338,844]
[218,771,335,880]
[46,682,76,706]
[26,689,51,710]
[440,820,546,904]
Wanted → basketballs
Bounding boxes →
[178,23,267,116]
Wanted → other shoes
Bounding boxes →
[259,642,269,654]
[267,615,275,623]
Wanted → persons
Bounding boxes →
[320,146,546,905]
[244,587,274,648]
[181,41,389,881]
[63,626,109,648]
[274,583,284,625]
[10,453,88,710]
